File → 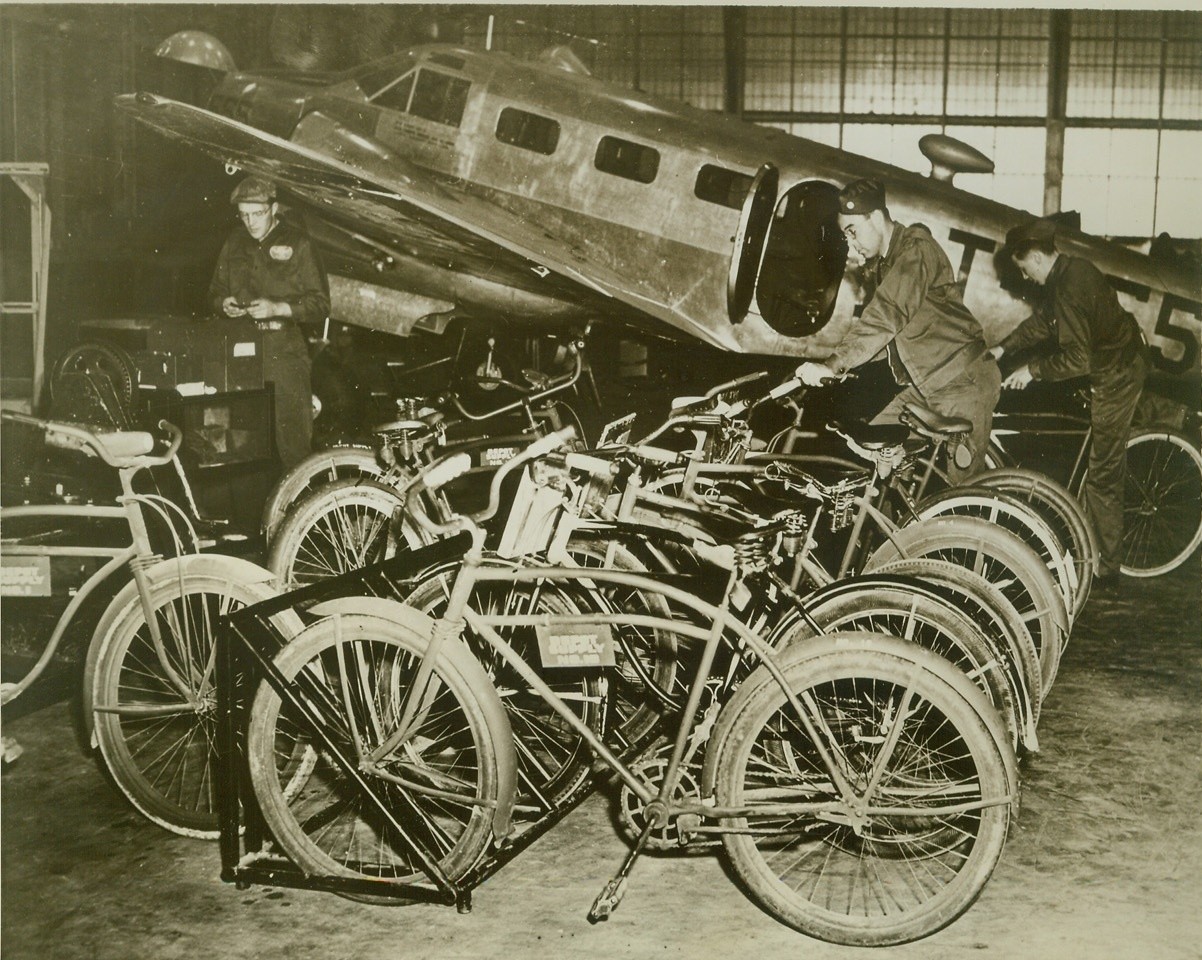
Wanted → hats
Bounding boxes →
[837,178,886,215]
[230,175,277,204]
[1003,217,1057,253]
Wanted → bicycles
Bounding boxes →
[2,405,324,839]
[243,338,1200,948]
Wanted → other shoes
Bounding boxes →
[1092,573,1121,588]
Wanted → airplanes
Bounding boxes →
[116,41,1202,411]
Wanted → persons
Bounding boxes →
[796,178,1002,491]
[206,176,331,474]
[988,219,1151,588]
